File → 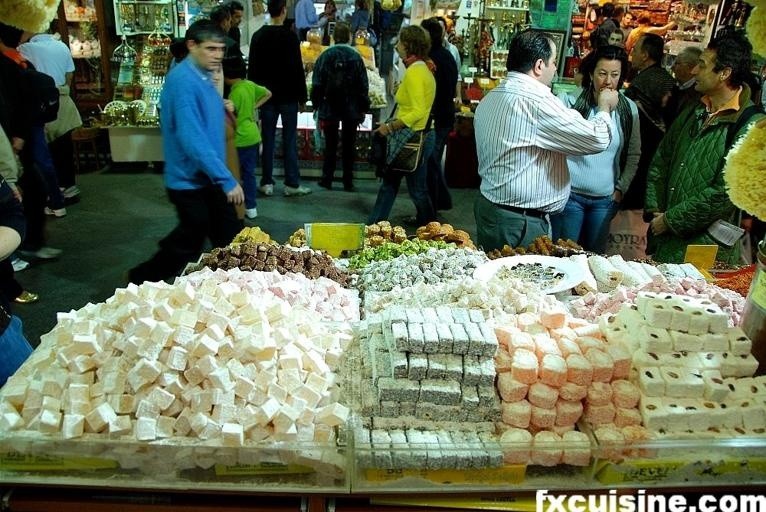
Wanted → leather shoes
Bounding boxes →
[318,179,333,190]
[344,185,355,191]
[403,217,419,226]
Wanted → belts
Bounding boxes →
[497,205,547,219]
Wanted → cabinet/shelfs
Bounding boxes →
[49,1,111,108]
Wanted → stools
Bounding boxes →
[71,129,107,172]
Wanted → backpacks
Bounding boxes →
[323,56,370,126]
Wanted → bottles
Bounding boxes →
[305,25,321,52]
[354,25,370,51]
[448,12,527,81]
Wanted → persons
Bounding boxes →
[120,0,766,284]
[0,0,82,390]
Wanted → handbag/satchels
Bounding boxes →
[370,128,426,175]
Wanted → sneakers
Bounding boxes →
[11,258,31,273]
[14,289,41,304]
[44,206,68,218]
[244,207,259,221]
[256,184,275,196]
[61,187,80,199]
[20,247,65,260]
[283,185,312,196]
